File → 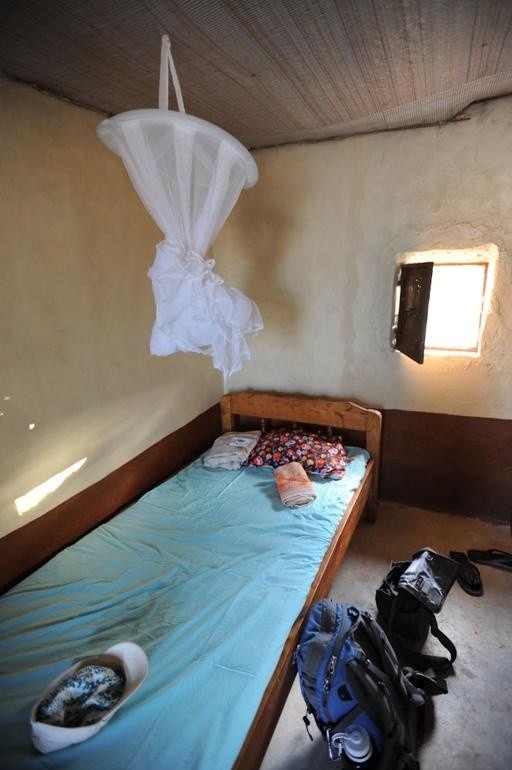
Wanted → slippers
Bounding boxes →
[467,549,512,572]
[449,551,483,597]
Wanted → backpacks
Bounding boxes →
[291,598,448,770]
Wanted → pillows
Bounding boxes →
[237,426,356,482]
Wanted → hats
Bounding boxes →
[29,641,148,753]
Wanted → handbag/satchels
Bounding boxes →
[375,545,462,664]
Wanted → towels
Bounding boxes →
[272,461,317,510]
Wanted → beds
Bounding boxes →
[2,388,385,770]
